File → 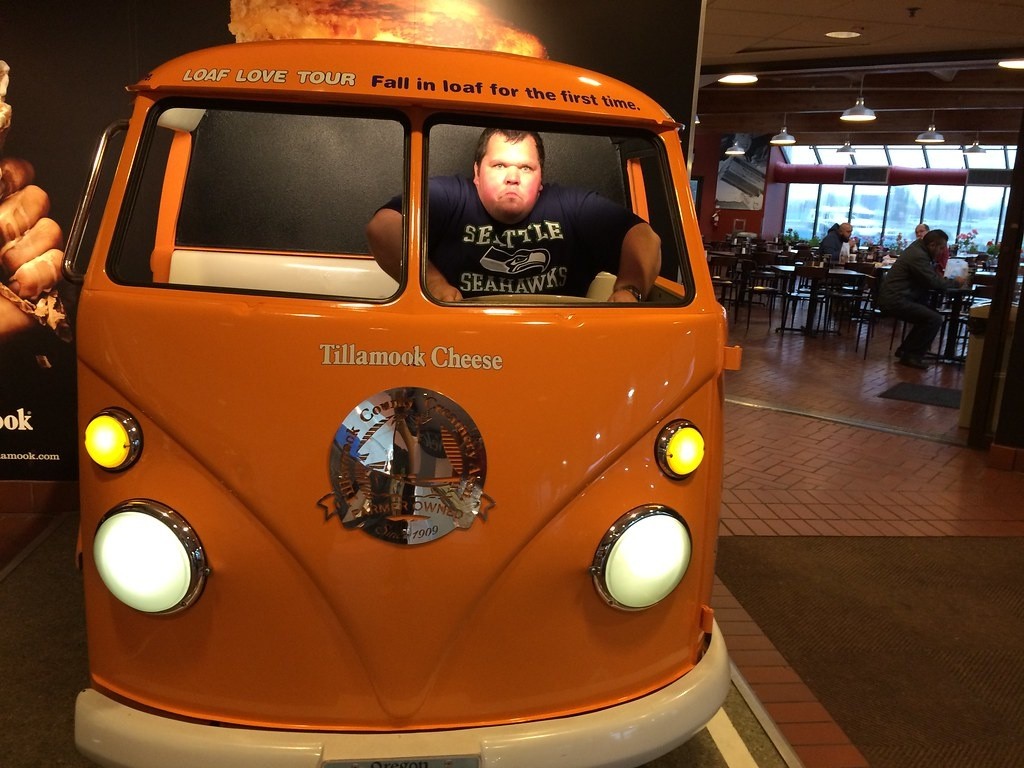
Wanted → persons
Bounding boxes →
[0,61,66,333]
[366,128,661,302]
[915,224,949,277]
[819,223,858,265]
[878,229,966,369]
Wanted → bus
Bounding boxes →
[48,0,743,768]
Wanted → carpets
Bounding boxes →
[877,380,962,409]
[714,535,1024,768]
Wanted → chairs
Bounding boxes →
[702,236,1024,368]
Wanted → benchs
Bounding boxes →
[167,246,614,302]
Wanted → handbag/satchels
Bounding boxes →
[944,259,969,288]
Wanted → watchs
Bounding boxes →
[622,285,645,302]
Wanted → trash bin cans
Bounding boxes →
[957,301,1019,432]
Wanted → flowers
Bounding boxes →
[779,227,980,253]
[987,240,1001,259]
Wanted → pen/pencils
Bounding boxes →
[951,275,956,277]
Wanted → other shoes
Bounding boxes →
[900,354,930,369]
[895,347,904,357]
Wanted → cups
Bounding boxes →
[849,254,855,261]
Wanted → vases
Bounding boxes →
[989,259,998,265]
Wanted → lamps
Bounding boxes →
[840,74,876,121]
[915,110,946,143]
[724,140,745,155]
[770,113,796,145]
[837,132,856,153]
[964,133,986,154]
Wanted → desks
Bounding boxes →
[950,254,978,258]
[708,250,735,277]
[766,248,799,253]
[924,287,972,362]
[772,266,868,339]
